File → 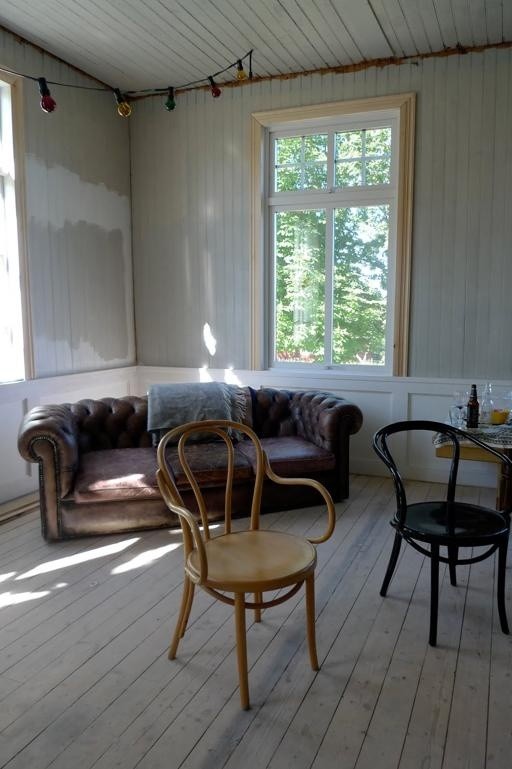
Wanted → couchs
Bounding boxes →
[16,386,364,541]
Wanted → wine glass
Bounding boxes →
[452,392,468,427]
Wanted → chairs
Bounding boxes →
[155,419,339,712]
[373,420,512,646]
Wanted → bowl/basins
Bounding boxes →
[492,410,509,424]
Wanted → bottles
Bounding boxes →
[467,383,493,428]
